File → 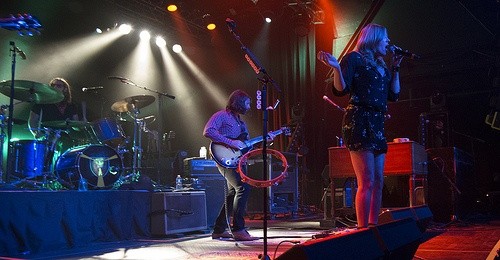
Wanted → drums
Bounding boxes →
[8,138,51,177]
[84,117,127,151]
[53,143,123,190]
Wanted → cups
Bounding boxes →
[78,179,88,191]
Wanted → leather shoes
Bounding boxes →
[212,231,232,238]
[233,232,253,240]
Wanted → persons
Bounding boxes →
[28,76,88,180]
[203,89,276,243]
[316,23,408,232]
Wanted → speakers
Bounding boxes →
[150,174,225,236]
[275,204,432,260]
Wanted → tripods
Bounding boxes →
[120,102,157,188]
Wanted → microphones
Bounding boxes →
[389,46,415,58]
[82,86,103,92]
[323,95,336,106]
[108,75,127,80]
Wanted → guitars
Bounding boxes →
[285,122,301,153]
[209,126,291,170]
[0,13,46,37]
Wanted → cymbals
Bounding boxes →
[0,79,66,104]
[110,94,155,113]
[235,148,290,188]
[41,120,90,129]
[4,116,28,125]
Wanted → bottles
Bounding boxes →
[176,175,183,190]
[200,146,207,160]
[338,138,343,147]
